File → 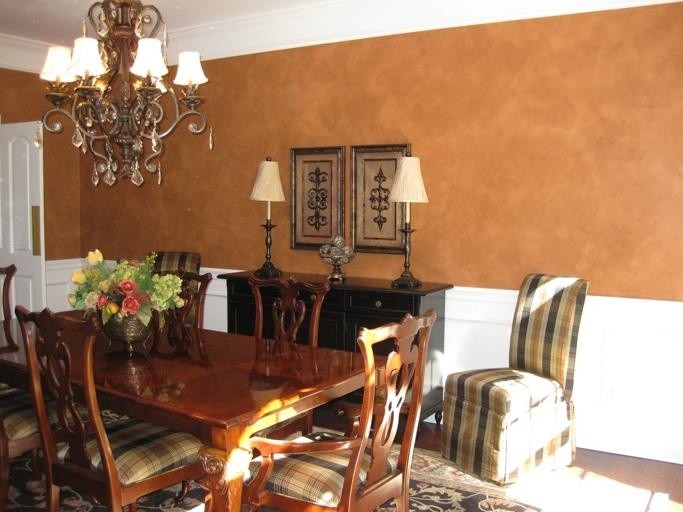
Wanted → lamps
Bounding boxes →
[249,156,286,279]
[387,157,430,288]
[39,0,214,188]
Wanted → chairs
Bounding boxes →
[442,272,590,487]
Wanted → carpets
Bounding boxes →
[0,375,669,512]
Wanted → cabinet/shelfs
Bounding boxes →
[217,269,455,404]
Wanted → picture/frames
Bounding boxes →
[350,143,411,255]
[290,145,346,251]
